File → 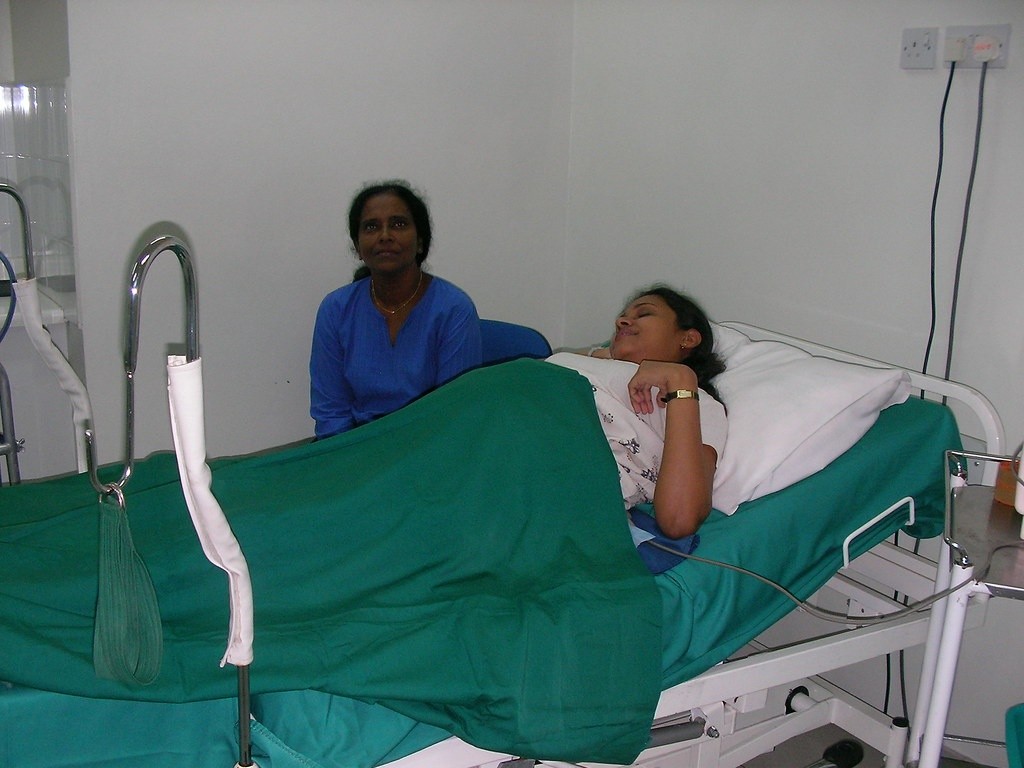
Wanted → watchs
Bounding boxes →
[661,389,699,404]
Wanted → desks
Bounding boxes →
[907,449,1024,768]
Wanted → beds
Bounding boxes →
[0,320,1001,768]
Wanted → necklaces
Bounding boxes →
[371,273,422,314]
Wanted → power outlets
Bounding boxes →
[943,24,1012,68]
[900,26,937,71]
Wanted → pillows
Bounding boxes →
[706,320,911,515]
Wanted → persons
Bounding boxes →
[540,286,728,541]
[308,184,483,439]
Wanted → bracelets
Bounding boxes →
[588,348,596,357]
